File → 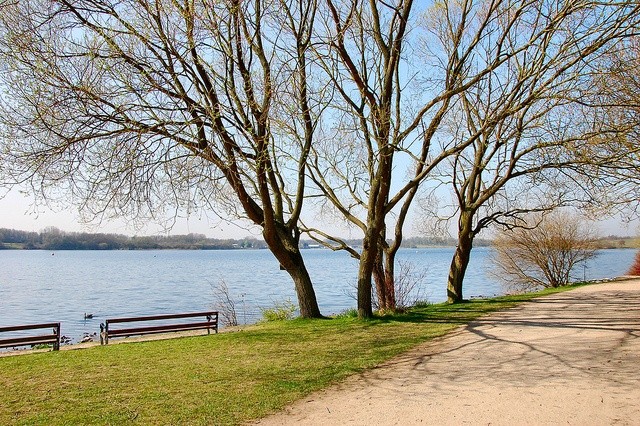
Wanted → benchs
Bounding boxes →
[0,323,61,352]
[99,311,218,345]
